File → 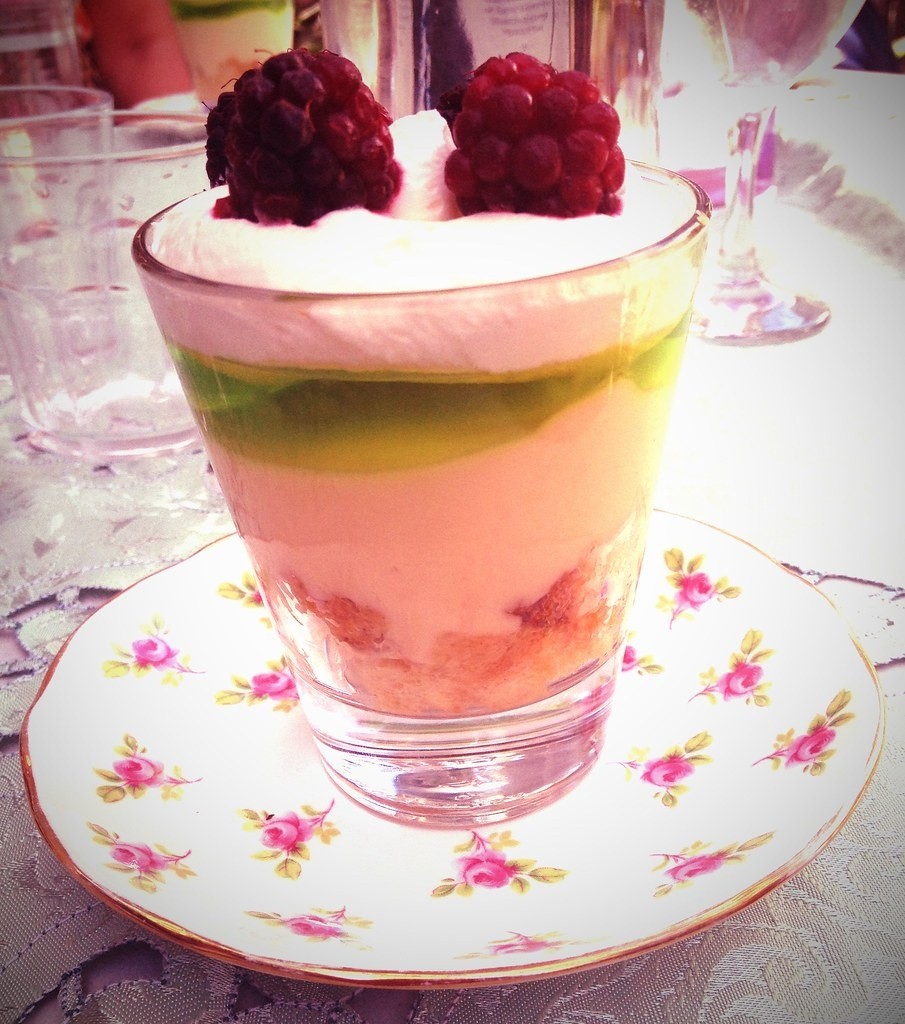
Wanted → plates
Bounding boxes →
[19,507,888,991]
[132,92,205,113]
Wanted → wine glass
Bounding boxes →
[659,0,864,344]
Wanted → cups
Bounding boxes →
[129,159,712,829]
[0,84,208,468]
[0,3,80,86]
[168,0,294,105]
